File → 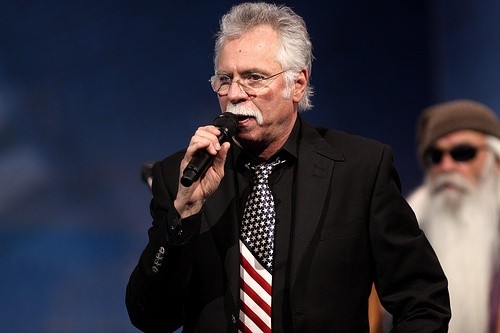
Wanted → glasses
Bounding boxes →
[424,144,488,164]
[208,68,293,93]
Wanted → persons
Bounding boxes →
[124,2,452,333]
[369,99,500,333]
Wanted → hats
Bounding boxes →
[411,100,500,173]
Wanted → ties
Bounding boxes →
[237,156,286,333]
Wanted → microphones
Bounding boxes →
[180,111,240,188]
